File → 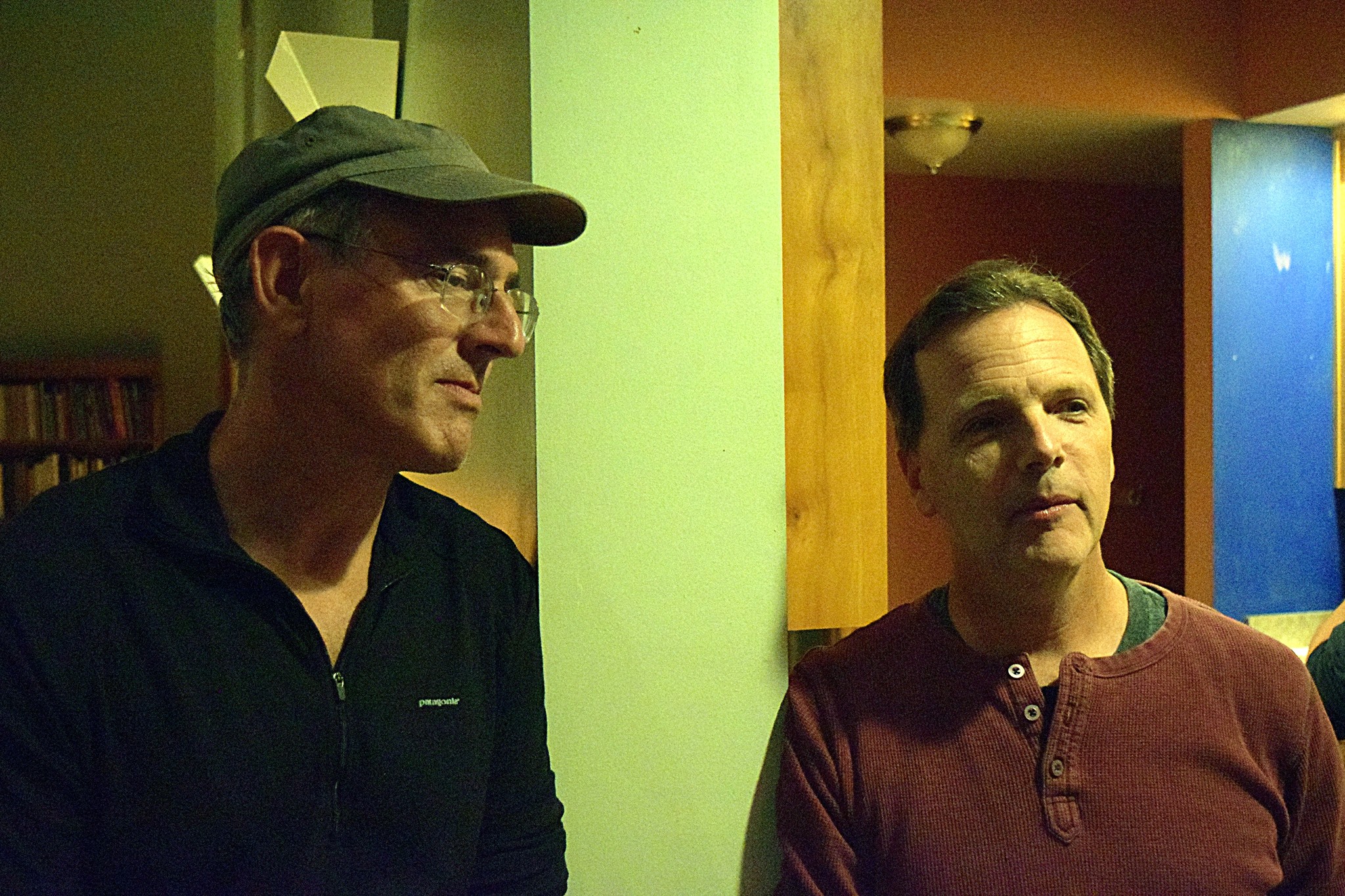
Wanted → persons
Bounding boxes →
[0,106,571,895]
[773,256,1345,896]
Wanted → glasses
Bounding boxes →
[295,227,540,346]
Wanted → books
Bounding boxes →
[0,369,159,511]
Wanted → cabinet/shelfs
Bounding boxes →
[0,351,164,512]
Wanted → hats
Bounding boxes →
[210,105,587,294]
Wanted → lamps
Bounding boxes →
[884,110,983,177]
